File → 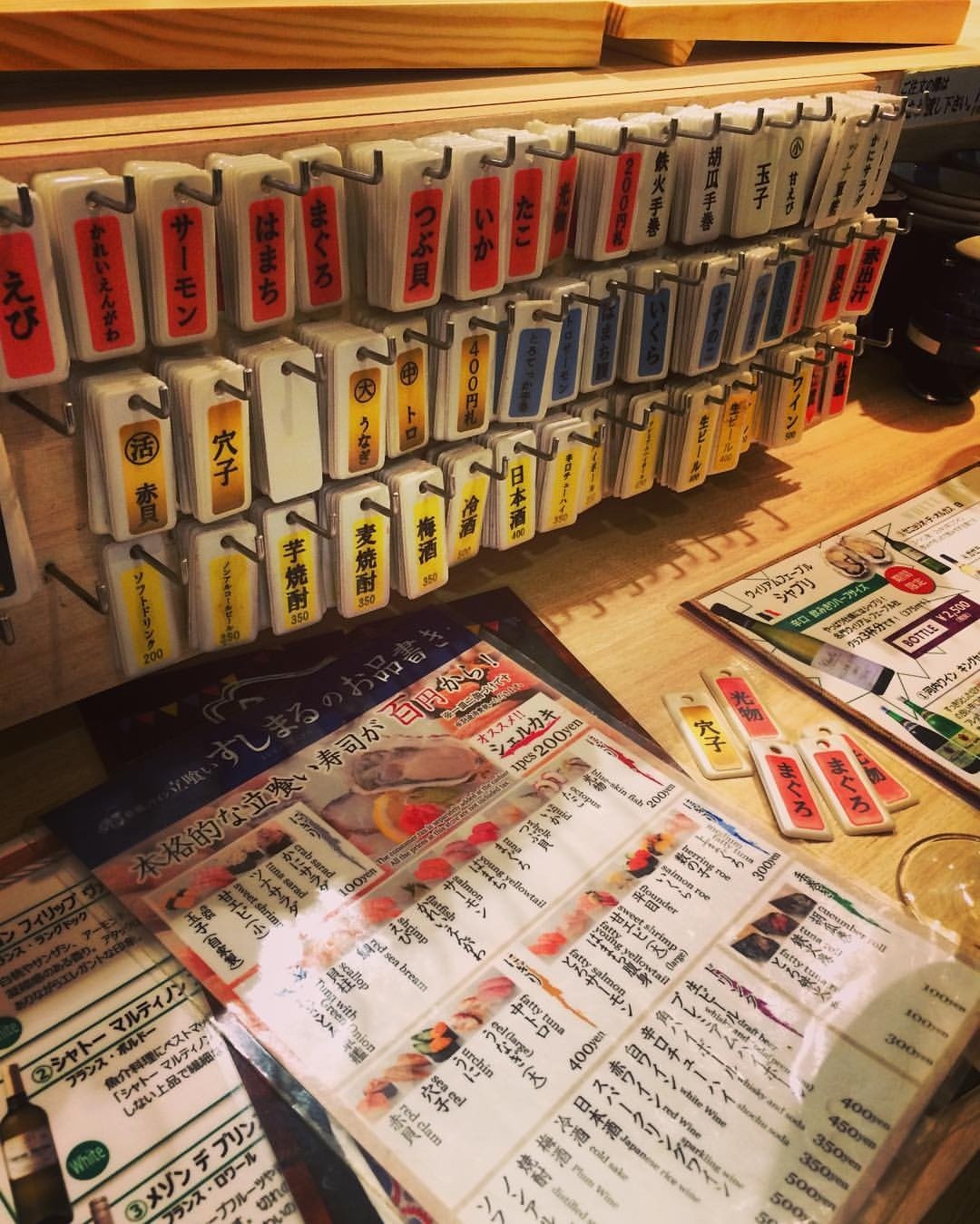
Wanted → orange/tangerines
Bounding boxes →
[374,788,416,841]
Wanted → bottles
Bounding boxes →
[898,695,979,757]
[0,1063,75,1224]
[710,601,893,696]
[872,531,951,575]
[940,554,980,581]
[881,706,980,774]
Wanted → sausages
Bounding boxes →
[475,974,512,998]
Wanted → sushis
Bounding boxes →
[409,1021,460,1061]
[623,848,658,877]
